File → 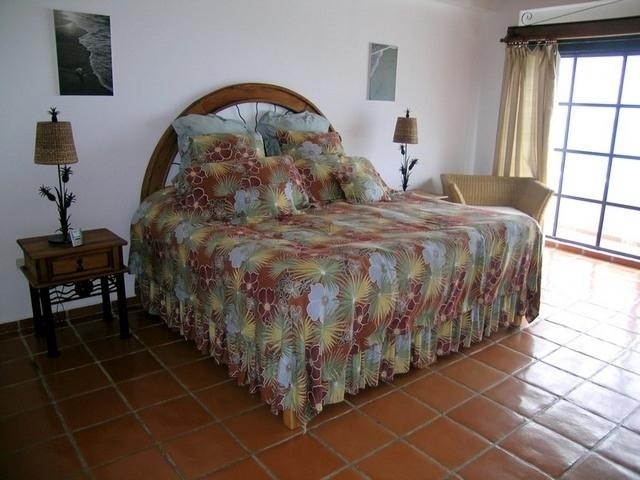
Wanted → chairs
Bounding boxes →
[441,174,553,226]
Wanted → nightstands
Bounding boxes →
[17,225,130,359]
[412,188,448,200]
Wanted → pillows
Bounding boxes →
[275,126,344,160]
[226,182,297,220]
[257,109,331,155]
[172,155,312,218]
[171,115,253,168]
[295,154,348,202]
[188,132,264,165]
[331,156,394,203]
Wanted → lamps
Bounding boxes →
[389,107,420,191]
[32,108,78,246]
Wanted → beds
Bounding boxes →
[124,82,544,435]
